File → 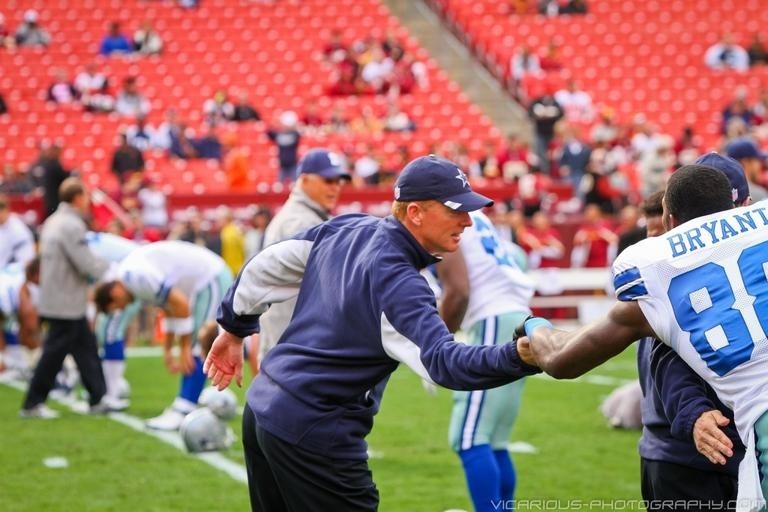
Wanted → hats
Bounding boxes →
[300,150,351,181]
[728,141,767,159]
[695,153,753,204]
[394,154,494,213]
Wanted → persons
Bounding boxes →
[0,0,768,512]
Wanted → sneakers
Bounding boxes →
[90,395,129,412]
[23,403,59,418]
[144,407,189,431]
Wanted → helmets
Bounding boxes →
[180,384,238,453]
